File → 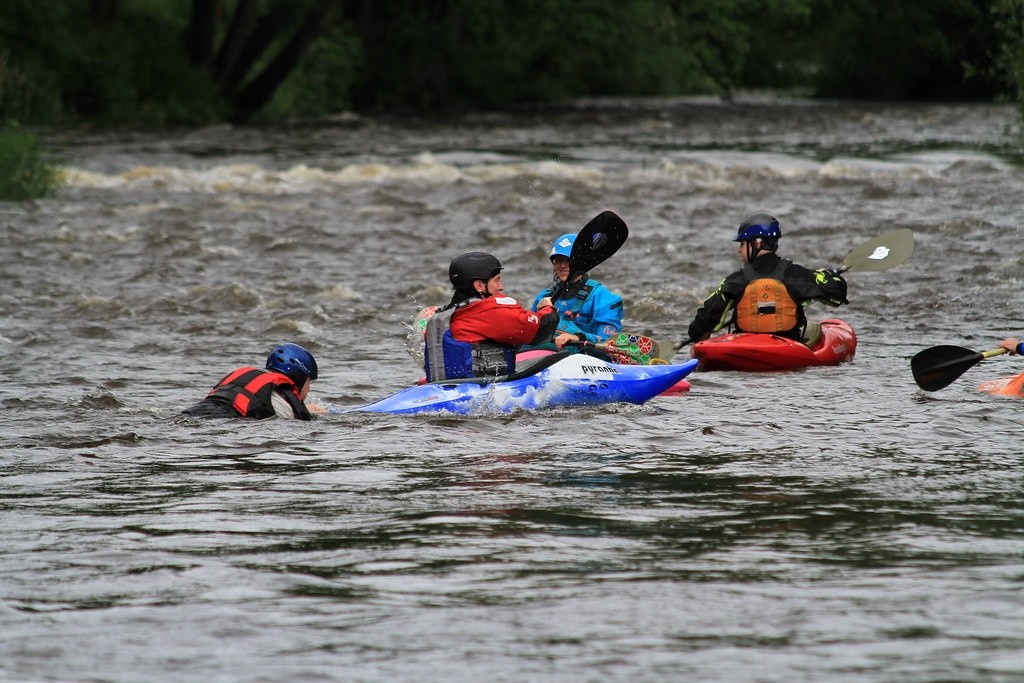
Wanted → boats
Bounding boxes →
[690,319,857,372]
[330,350,699,415]
[979,371,1024,403]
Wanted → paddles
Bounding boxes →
[646,224,916,366]
[411,304,660,366]
[516,209,631,353]
[910,344,1009,393]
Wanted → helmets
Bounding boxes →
[449,252,504,289]
[732,214,783,242]
[549,234,577,261]
[265,343,318,379]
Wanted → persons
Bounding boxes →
[529,233,625,363]
[179,342,329,420]
[687,213,848,343]
[998,338,1024,356]
[423,251,560,385]
